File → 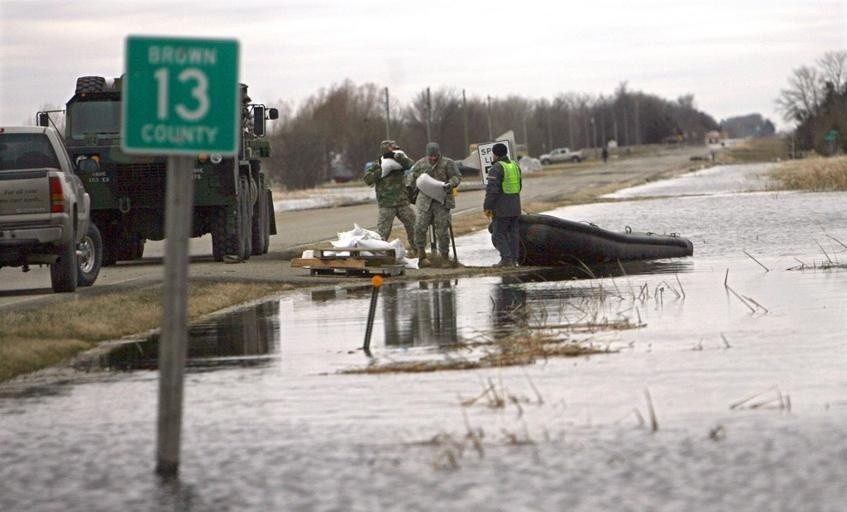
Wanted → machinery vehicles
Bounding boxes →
[34,72,282,266]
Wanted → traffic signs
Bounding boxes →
[477,138,514,187]
[119,32,245,158]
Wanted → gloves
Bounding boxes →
[484,209,494,220]
[443,182,452,193]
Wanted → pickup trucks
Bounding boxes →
[0,123,103,300]
[539,146,585,167]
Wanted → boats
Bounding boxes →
[519,209,696,267]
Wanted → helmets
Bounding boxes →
[380,141,397,153]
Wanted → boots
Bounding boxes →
[439,253,449,267]
[493,257,519,267]
[408,247,431,266]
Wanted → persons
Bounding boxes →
[362,139,428,259]
[483,143,522,268]
[405,142,462,269]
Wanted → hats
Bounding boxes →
[492,144,507,156]
[426,143,440,155]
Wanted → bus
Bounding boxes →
[468,143,529,161]
